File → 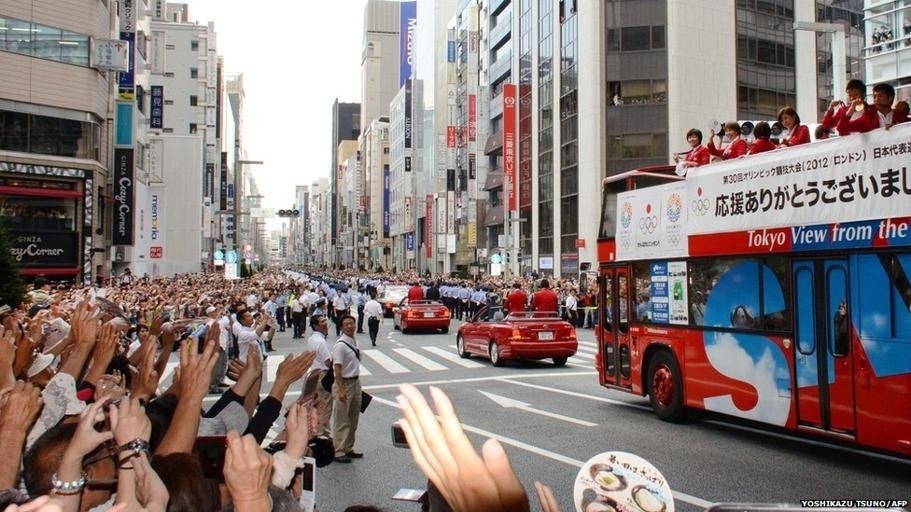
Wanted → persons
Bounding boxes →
[871,17,910,51]
[1,264,710,512]
[681,77,911,168]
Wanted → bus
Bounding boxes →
[594,113,911,471]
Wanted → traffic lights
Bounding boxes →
[277,208,300,216]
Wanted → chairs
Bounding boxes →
[489,312,504,322]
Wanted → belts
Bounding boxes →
[344,376,358,381]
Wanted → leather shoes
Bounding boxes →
[336,455,352,463]
[346,450,363,457]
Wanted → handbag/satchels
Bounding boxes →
[322,366,334,392]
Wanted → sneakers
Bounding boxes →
[210,383,228,394]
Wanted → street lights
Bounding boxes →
[486,171,513,283]
[791,19,847,106]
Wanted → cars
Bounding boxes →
[377,289,411,318]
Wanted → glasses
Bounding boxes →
[84,447,118,470]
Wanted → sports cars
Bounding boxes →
[456,303,580,368]
[391,295,453,335]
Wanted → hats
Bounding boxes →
[359,287,366,293]
[23,352,54,376]
[443,281,494,291]
[206,307,217,314]
[315,298,325,306]
[329,282,348,292]
[198,400,250,439]
[42,318,71,352]
